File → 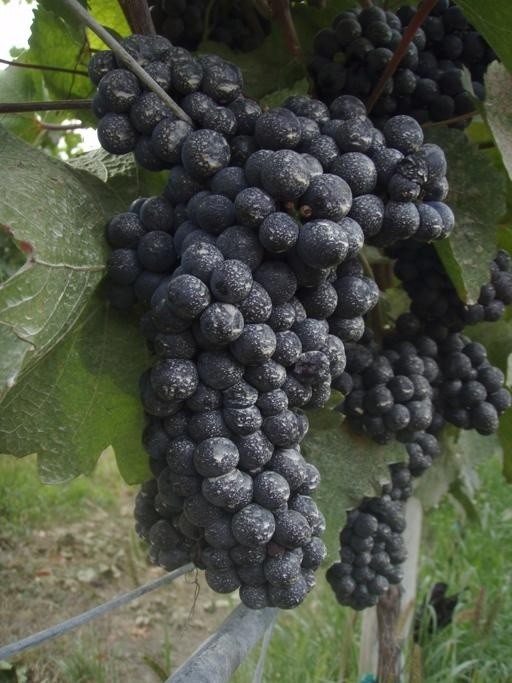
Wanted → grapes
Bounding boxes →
[87,0,512,611]
